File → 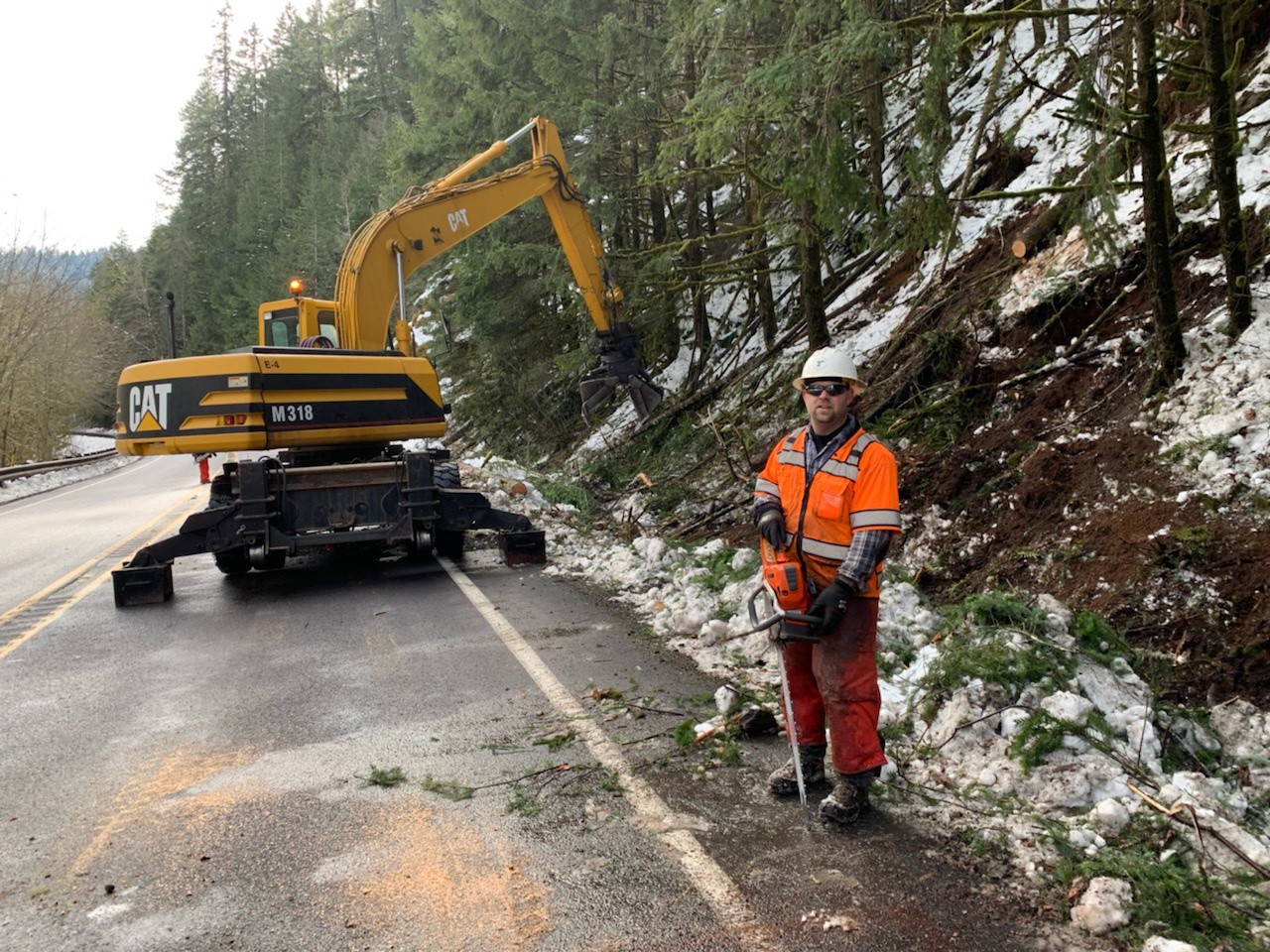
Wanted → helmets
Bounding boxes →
[791,347,868,396]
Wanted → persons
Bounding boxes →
[756,346,899,831]
[193,452,216,484]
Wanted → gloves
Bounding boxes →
[807,581,855,635]
[755,506,787,550]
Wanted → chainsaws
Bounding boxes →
[747,533,823,831]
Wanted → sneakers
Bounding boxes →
[769,748,825,789]
[818,782,868,821]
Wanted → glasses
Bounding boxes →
[801,383,850,396]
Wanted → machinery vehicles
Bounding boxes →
[111,116,664,607]
